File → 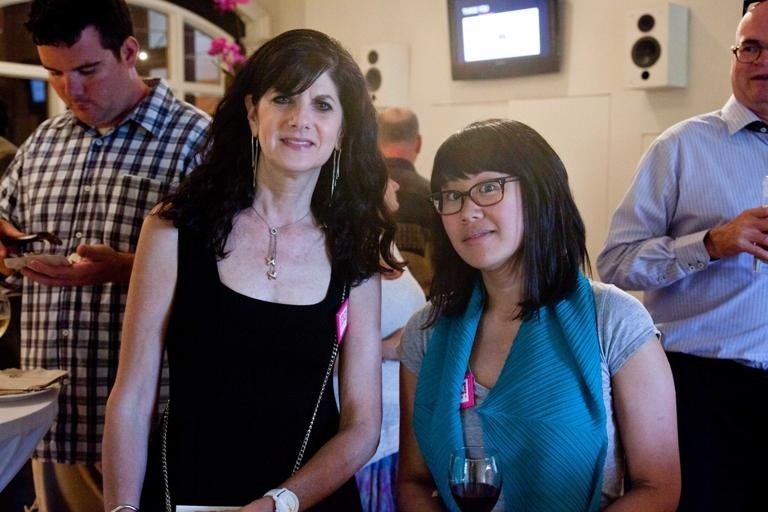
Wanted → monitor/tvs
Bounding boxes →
[448,0,560,81]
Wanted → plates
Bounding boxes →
[0,385,54,404]
[3,255,65,269]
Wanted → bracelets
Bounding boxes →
[107,502,140,512]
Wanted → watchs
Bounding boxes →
[262,485,302,512]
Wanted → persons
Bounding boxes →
[596,0,768,512]
[392,114,684,512]
[99,27,411,512]
[333,137,427,512]
[0,1,215,512]
[374,100,442,294]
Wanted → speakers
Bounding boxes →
[357,43,409,111]
[623,2,689,90]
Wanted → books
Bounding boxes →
[0,365,68,395]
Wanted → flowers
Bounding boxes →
[207,0,252,84]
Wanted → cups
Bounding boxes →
[0,295,11,339]
[448,446,502,512]
[754,175,768,272]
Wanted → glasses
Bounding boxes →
[730,43,768,65]
[425,175,520,216]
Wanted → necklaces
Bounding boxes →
[249,202,313,282]
